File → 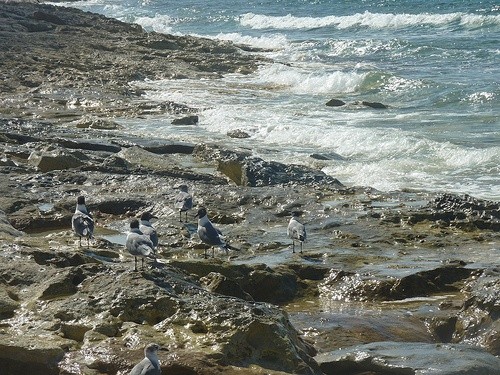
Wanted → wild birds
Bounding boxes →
[138,212,159,249]
[128,343,169,375]
[72,196,95,248]
[126,220,159,263]
[173,184,193,222]
[193,207,240,250]
[286,210,306,246]
